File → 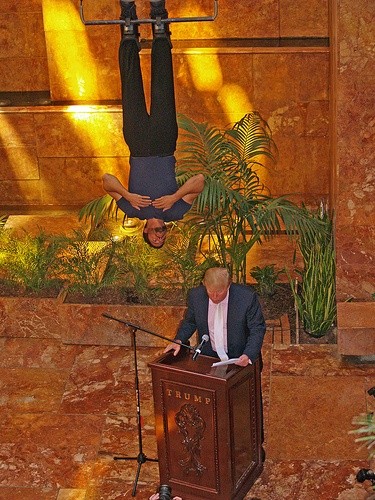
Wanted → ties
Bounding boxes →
[214,303,228,362]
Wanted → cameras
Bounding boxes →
[156,484,173,500]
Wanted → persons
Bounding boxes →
[102,1,206,249]
[164,267,266,465]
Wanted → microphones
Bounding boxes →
[191,334,209,360]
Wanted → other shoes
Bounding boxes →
[120,0,135,9]
[150,0,164,10]
[261,447,265,462]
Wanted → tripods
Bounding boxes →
[101,312,195,496]
